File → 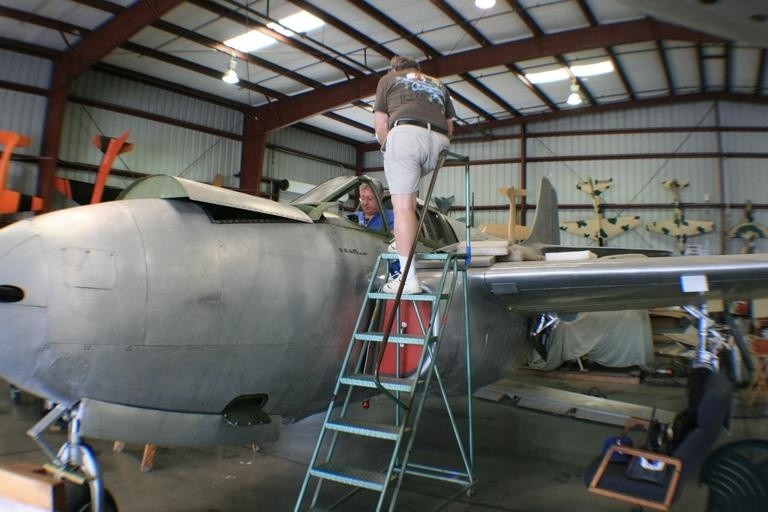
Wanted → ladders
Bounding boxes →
[293,251,481,512]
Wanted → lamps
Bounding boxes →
[221,58,239,84]
[566,84,582,105]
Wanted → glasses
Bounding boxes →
[358,197,376,201]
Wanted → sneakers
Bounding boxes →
[382,275,422,294]
[387,238,398,255]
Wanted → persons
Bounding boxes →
[373,56,455,295]
[346,180,394,233]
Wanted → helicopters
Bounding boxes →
[431,197,473,227]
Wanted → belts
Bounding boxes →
[389,119,451,137]
